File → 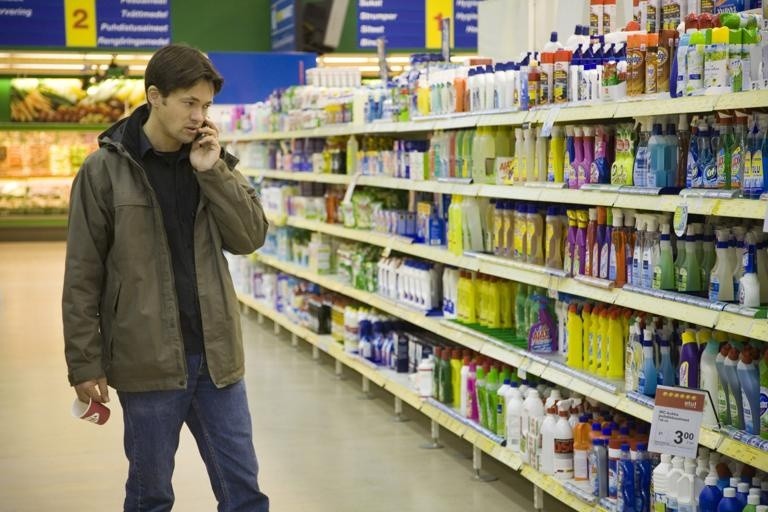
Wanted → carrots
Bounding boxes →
[10,91,50,121]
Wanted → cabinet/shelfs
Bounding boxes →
[219,88,768,512]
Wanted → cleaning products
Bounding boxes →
[230,15,768,512]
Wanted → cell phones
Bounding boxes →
[194,116,210,141]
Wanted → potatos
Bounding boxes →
[41,103,121,122]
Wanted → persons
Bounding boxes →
[60,42,274,512]
